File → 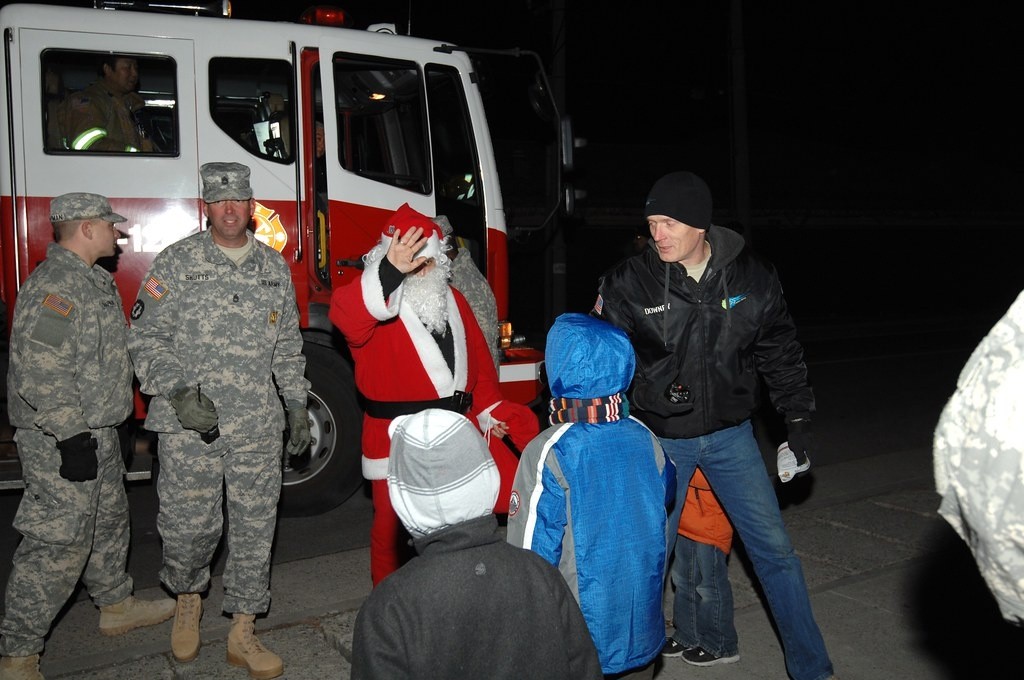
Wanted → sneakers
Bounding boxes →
[662,638,740,665]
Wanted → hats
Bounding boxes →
[48,193,127,224]
[382,205,445,261]
[643,169,712,229]
[201,162,254,203]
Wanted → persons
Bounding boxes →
[934,289,1024,626]
[124,162,313,680]
[312,123,507,586]
[58,54,160,156]
[590,171,834,680]
[503,314,672,680]
[0,192,175,680]
[356,408,607,680]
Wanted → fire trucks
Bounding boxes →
[0,0,575,516]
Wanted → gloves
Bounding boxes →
[171,383,218,434]
[786,418,825,470]
[56,433,99,482]
[285,409,312,455]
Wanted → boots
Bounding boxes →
[99,596,177,636]
[172,595,202,662]
[0,654,43,680]
[228,612,284,678]
